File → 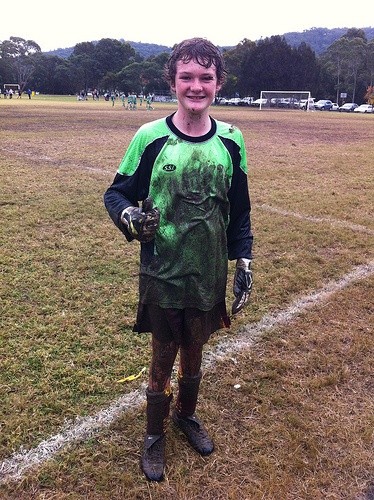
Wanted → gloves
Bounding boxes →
[120,205,161,244]
[231,256,253,316]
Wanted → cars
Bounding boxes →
[252,98,270,107]
[211,97,227,106]
[266,97,316,110]
[338,103,359,112]
[225,97,241,106]
[354,104,374,113]
[329,102,340,111]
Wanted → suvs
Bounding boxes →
[238,96,256,106]
[313,100,333,111]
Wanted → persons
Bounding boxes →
[104,37,253,480]
[139,94,143,107]
[126,91,137,110]
[27,89,31,99]
[4,89,13,99]
[111,92,114,106]
[95,91,99,101]
[18,89,21,98]
[104,92,109,101]
[144,94,154,110]
[122,92,125,107]
[80,89,85,101]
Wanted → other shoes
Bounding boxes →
[174,408,214,456]
[141,431,165,481]
